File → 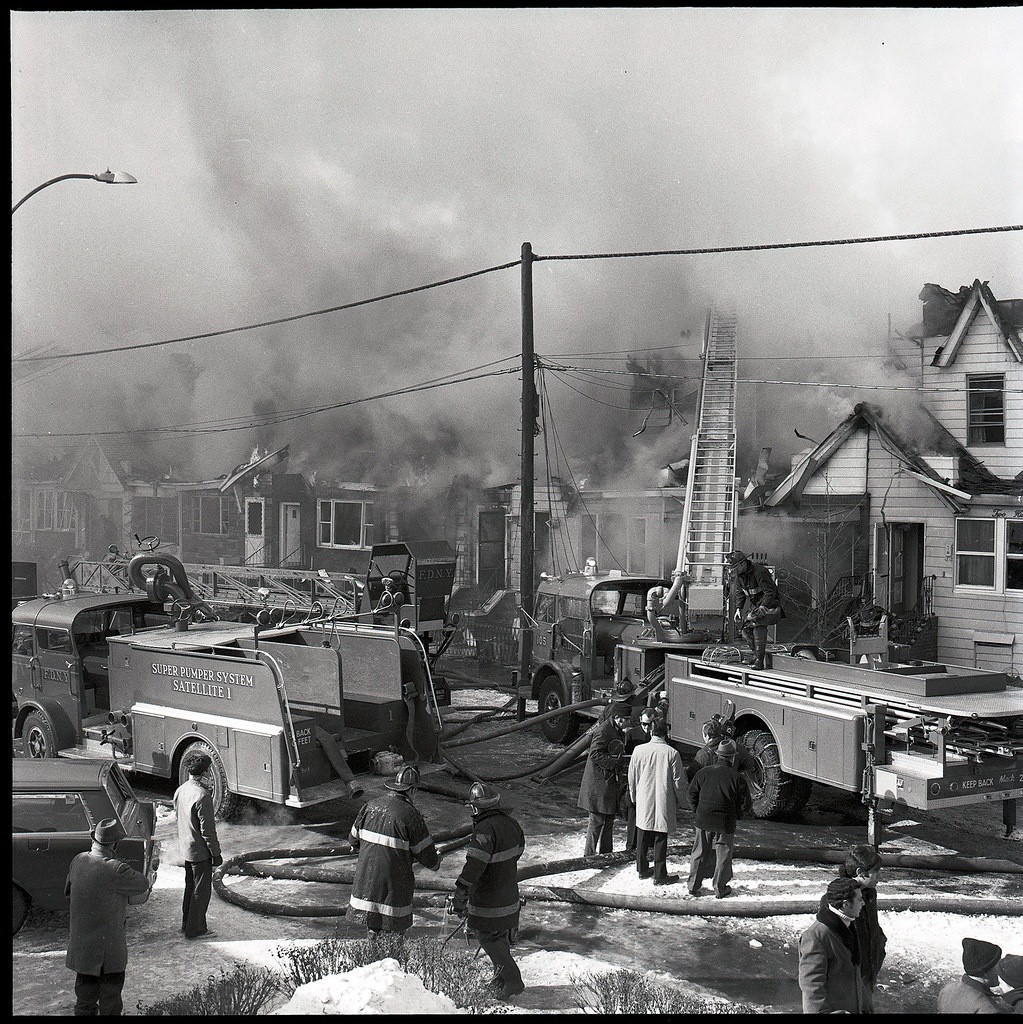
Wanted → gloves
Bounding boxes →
[212,856,223,868]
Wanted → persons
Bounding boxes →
[626,707,672,853]
[102,515,117,545]
[453,781,527,1001]
[798,878,875,1014]
[575,702,633,859]
[62,816,149,1016]
[937,938,1023,1014]
[347,765,444,946]
[724,549,788,670]
[687,711,754,901]
[627,717,687,885]
[173,752,223,941]
[839,846,887,984]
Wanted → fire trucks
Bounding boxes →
[12,532,461,823]
[516,298,1023,851]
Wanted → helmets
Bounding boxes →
[383,765,420,791]
[465,783,501,808]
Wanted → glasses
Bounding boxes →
[620,716,631,721]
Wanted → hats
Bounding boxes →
[995,954,1023,989]
[726,550,754,567]
[90,818,127,844]
[637,708,659,724]
[713,740,739,756]
[962,937,1002,976]
[612,702,634,716]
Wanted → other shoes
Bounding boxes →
[654,875,679,886]
[188,929,217,939]
[751,658,765,670]
[497,978,526,1000]
[716,885,730,899]
[639,866,655,880]
[741,654,756,664]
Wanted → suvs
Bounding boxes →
[12,758,160,940]
